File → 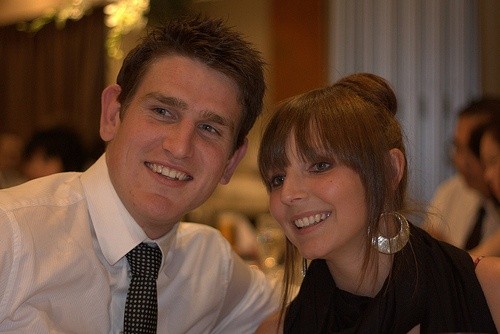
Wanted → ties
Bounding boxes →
[122,242,163,334]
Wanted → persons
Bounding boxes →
[0,7,299,334]
[424,95,500,258]
[23,122,84,178]
[257,68,500,334]
